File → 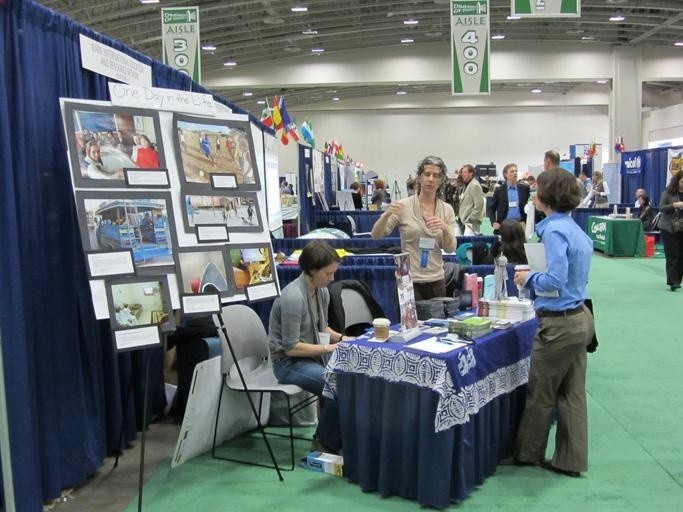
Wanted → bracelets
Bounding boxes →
[338,333,345,341]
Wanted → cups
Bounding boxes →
[371,318,390,341]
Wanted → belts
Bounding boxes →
[535,304,588,318]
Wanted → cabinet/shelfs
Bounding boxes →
[475,164,496,222]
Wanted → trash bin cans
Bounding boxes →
[644,236,655,257]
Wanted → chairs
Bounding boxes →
[330,280,383,338]
[346,215,372,237]
[644,212,662,250]
[212,304,323,473]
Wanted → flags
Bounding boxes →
[326,137,351,166]
[261,93,316,149]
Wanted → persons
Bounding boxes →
[372,180,385,206]
[267,240,357,456]
[446,165,484,235]
[113,213,153,237]
[349,182,363,212]
[280,180,294,195]
[499,168,594,476]
[242,217,253,225]
[488,164,530,229]
[652,170,683,291]
[575,172,593,208]
[497,252,509,298]
[634,189,646,218]
[198,131,236,168]
[236,258,248,271]
[76,127,160,180]
[247,205,254,223]
[592,170,610,208]
[634,195,652,231]
[542,151,573,217]
[493,220,533,301]
[370,155,457,323]
[180,129,187,152]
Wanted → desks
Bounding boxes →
[587,216,646,258]
[321,308,557,510]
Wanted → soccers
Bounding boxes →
[198,170,205,177]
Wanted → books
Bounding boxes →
[478,297,533,321]
[431,296,459,316]
[448,317,489,339]
[415,300,445,321]
